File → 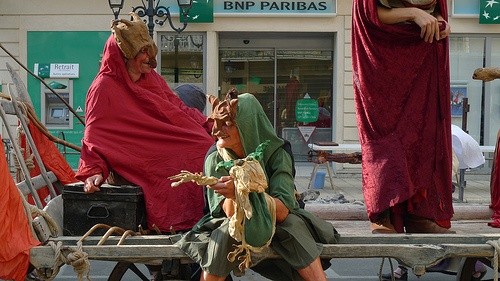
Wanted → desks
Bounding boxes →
[307,143,496,201]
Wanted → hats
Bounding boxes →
[175,84,206,115]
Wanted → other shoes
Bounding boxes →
[371,218,395,234]
[472,270,488,281]
[405,220,456,233]
[379,265,408,281]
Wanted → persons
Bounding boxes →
[351,0,456,233]
[381,153,487,281]
[200,88,329,281]
[75,12,215,232]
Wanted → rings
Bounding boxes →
[86,185,88,189]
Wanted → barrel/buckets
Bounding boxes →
[311,172,326,189]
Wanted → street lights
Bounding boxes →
[106,0,193,41]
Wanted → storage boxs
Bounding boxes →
[62,181,145,236]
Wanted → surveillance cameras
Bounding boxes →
[244,40,249,44]
